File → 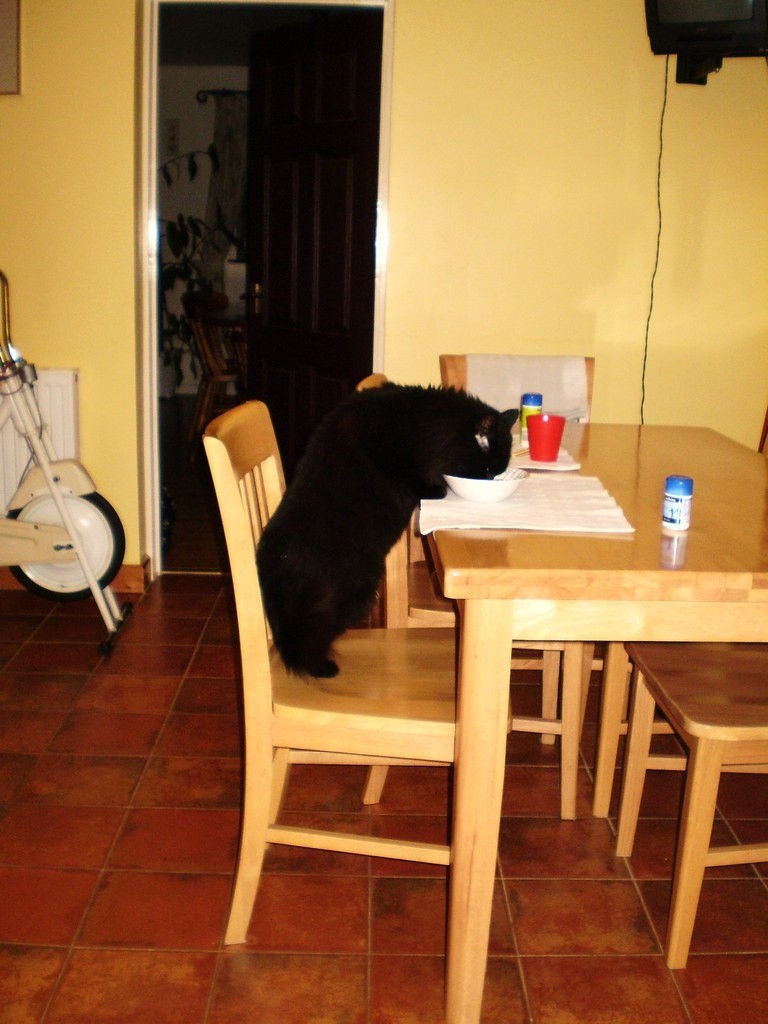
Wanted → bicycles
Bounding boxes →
[1,271,134,662]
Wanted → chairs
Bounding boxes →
[440,354,596,421]
[179,290,283,471]
[202,401,512,945]
[357,373,585,819]
[613,642,768,969]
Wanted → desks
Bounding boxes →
[419,422,768,1024]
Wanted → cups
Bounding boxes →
[526,414,566,462]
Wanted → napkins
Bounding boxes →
[508,434,582,471]
[420,475,637,536]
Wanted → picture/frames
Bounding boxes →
[0,0,22,96]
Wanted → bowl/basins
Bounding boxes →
[443,464,530,503]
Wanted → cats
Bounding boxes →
[252,381,520,678]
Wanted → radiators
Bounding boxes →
[0,366,80,519]
